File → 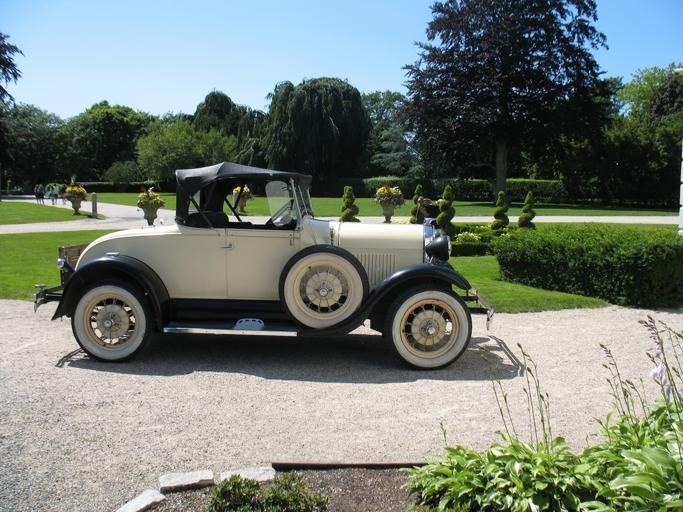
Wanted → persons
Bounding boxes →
[32,183,67,206]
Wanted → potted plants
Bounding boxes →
[232,186,250,215]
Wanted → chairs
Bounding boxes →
[184,210,227,228]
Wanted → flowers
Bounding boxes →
[136,188,165,208]
[374,186,404,208]
[62,186,88,201]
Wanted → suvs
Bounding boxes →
[27,159,495,371]
